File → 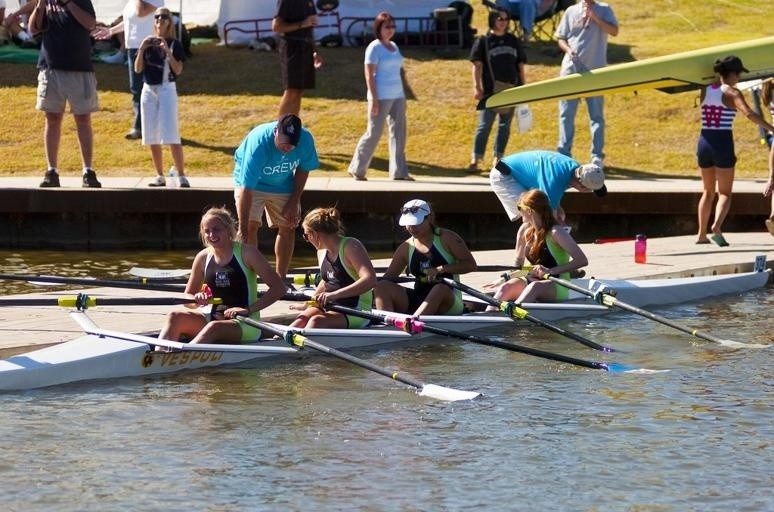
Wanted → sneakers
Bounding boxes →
[348,169,417,182]
[466,163,477,173]
[521,33,532,48]
[569,267,585,278]
[179,175,189,187]
[149,175,167,186]
[82,170,101,188]
[39,170,60,187]
[124,129,142,140]
[592,157,603,168]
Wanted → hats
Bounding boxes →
[278,113,300,147]
[721,55,749,74]
[577,163,607,198]
[398,199,429,226]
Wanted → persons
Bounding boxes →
[759,75,774,238]
[270,206,378,339]
[374,198,479,318]
[269,1,323,126]
[230,113,320,279]
[553,1,619,169]
[0,0,192,67]
[346,10,417,182]
[464,6,529,172]
[484,148,609,269]
[132,5,191,188]
[26,0,105,189]
[694,54,774,247]
[493,1,541,44]
[152,206,289,352]
[484,187,591,314]
[91,0,168,143]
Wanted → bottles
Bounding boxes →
[634,233,646,264]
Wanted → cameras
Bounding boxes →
[150,37,161,45]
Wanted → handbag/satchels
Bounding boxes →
[489,80,516,115]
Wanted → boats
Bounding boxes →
[1,268,771,393]
[471,29,773,114]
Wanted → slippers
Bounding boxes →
[764,218,774,234]
[696,232,728,246]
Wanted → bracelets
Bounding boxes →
[499,271,509,281]
[298,20,304,29]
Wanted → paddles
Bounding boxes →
[325,303,670,373]
[736,79,767,145]
[544,274,772,349]
[130,262,408,279]
[234,313,484,400]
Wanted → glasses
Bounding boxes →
[384,25,396,29]
[303,232,314,243]
[400,205,429,214]
[154,14,169,21]
[516,202,534,213]
[496,16,508,21]
